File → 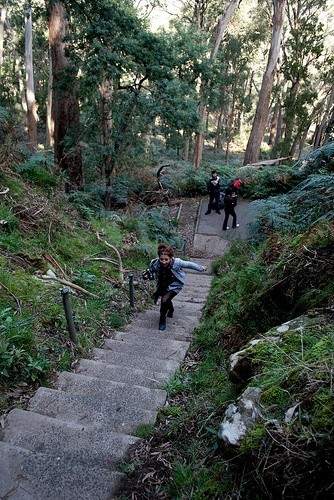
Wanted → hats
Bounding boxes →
[233,179,242,188]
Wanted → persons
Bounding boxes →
[204,170,221,215]
[222,178,244,231]
[142,243,208,330]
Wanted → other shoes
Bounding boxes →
[236,224,240,228]
[227,227,229,230]
[159,316,166,330]
[167,308,174,318]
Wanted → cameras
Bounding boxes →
[144,268,154,281]
